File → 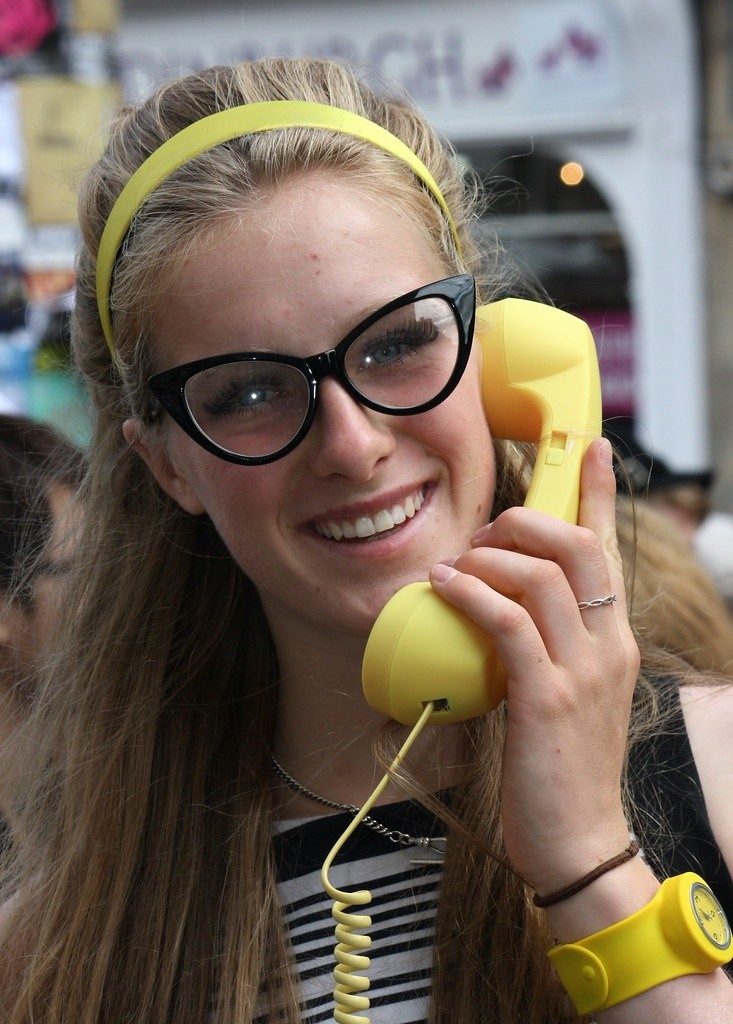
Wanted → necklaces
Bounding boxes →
[271,752,448,855]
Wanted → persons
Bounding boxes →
[0,55,733,1024]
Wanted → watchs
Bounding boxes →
[547,872,733,1016]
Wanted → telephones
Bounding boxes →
[361,294,613,732]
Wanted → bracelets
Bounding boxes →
[533,840,639,908]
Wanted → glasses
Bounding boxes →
[148,276,477,465]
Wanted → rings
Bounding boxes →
[577,594,617,610]
